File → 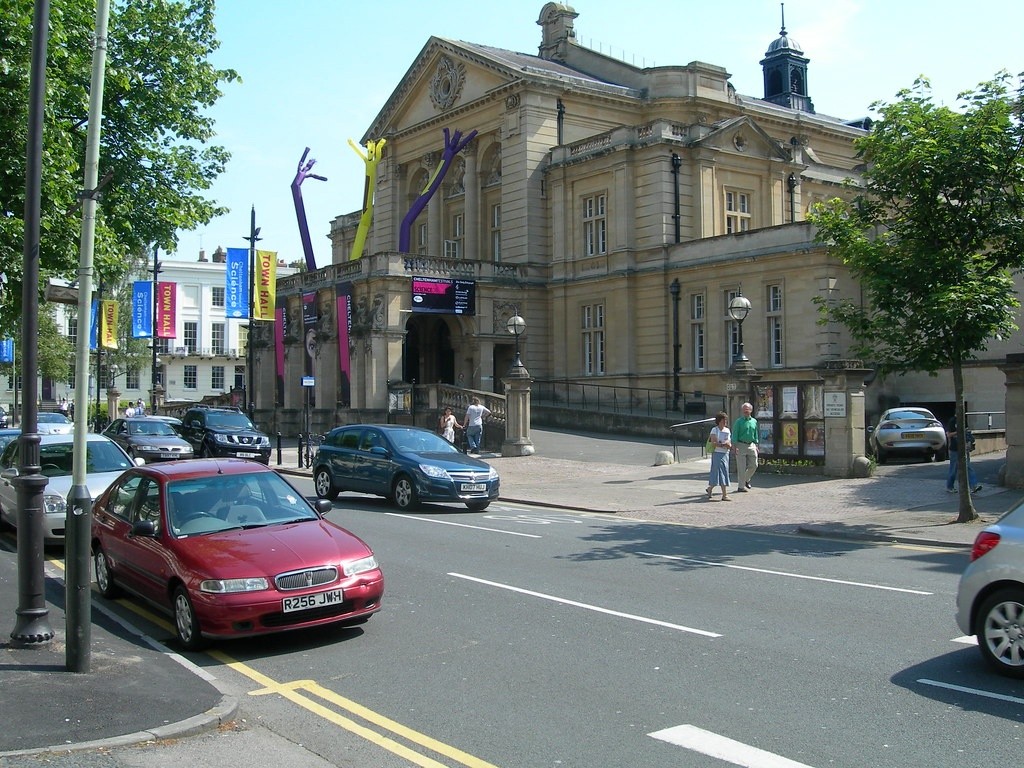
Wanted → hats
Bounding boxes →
[129,401,134,405]
[137,400,142,404]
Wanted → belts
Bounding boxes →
[738,440,754,444]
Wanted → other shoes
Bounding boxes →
[970,485,983,494]
[722,495,731,501]
[738,487,748,492]
[706,487,713,498]
[471,446,477,453]
[746,480,752,489]
[946,487,958,493]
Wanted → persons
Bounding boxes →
[946,412,982,494]
[440,407,463,444]
[70,403,74,422]
[706,411,732,501]
[463,397,491,453]
[70,399,75,406]
[731,403,759,492]
[60,398,68,418]
[125,398,146,418]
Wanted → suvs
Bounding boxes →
[179,404,272,466]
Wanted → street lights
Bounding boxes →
[148,270,164,414]
[244,237,264,422]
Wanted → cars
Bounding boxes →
[19,412,75,434]
[0,428,22,457]
[101,418,194,463]
[312,424,501,512]
[95,455,384,650]
[0,407,9,428]
[867,407,945,463]
[0,434,146,546]
[129,415,183,433]
[955,490,1024,677]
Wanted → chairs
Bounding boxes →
[208,479,274,519]
[150,492,188,534]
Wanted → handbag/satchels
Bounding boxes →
[436,416,445,435]
[705,427,718,453]
